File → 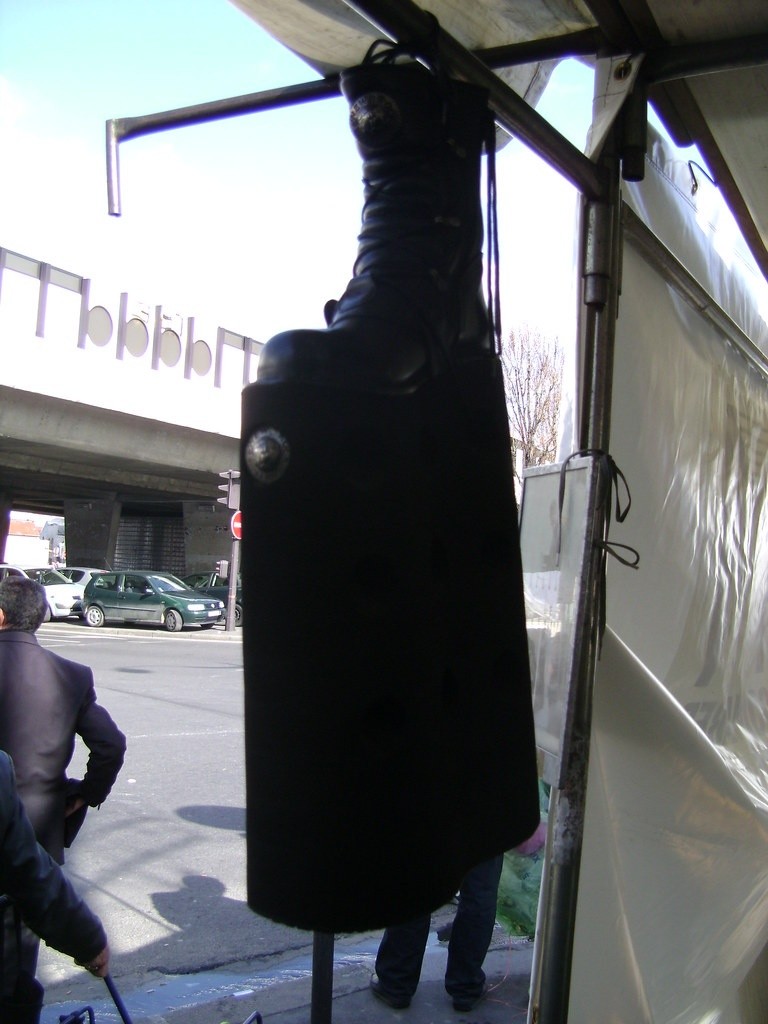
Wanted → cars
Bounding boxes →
[82,570,225,631]
[0,565,86,621]
[180,571,242,628]
[55,568,112,588]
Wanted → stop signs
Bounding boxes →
[231,511,243,541]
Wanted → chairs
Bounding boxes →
[126,580,141,594]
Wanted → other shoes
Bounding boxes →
[369,974,408,1009]
[456,980,487,1012]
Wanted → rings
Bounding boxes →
[85,965,90,969]
[95,966,98,970]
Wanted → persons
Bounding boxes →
[0,576,127,978]
[370,849,504,1012]
[0,495,110,1024]
